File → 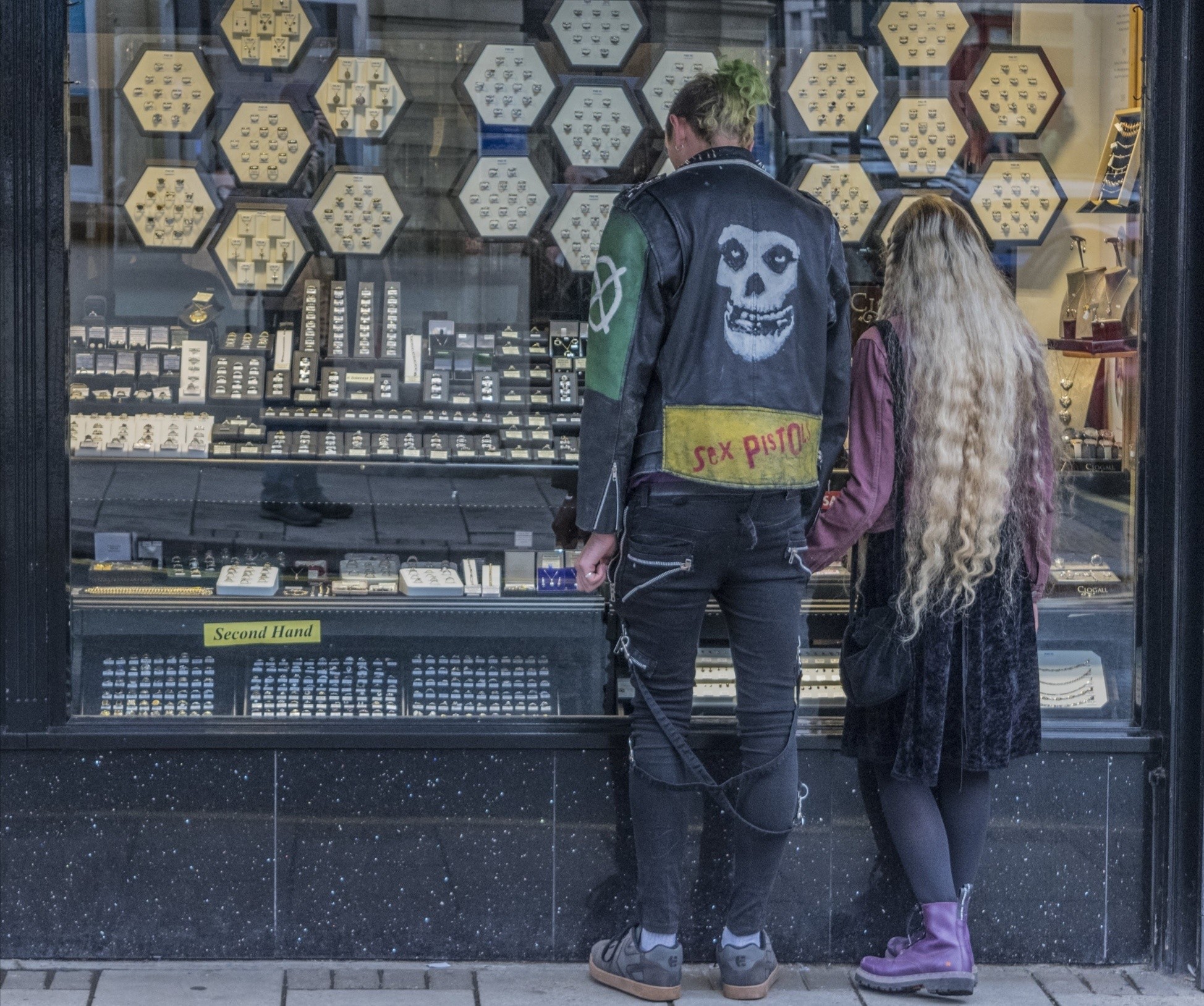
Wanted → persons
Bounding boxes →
[808,190,1062,996]
[572,62,851,1002]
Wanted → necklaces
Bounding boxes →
[234,0,296,55]
[468,551,577,598]
[1038,653,1095,707]
[231,215,288,287]
[1100,119,1138,196]
[1057,269,1130,423]
[330,61,390,128]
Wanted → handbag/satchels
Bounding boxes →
[838,604,918,705]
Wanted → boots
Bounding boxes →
[854,881,980,995]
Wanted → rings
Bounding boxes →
[584,572,592,579]
[132,62,299,238]
[688,643,845,695]
[68,285,584,461]
[81,540,552,718]
[468,0,701,261]
[325,185,390,241]
[800,9,1049,234]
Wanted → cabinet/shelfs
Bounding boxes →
[1047,107,1142,359]
[69,321,1133,718]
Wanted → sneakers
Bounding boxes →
[714,927,782,998]
[590,918,683,1001]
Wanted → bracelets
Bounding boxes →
[1053,552,1111,584]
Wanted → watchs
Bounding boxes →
[1060,426,1116,460]
